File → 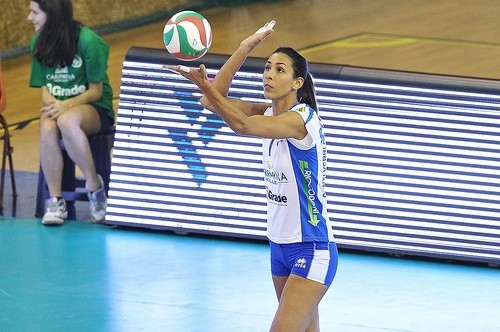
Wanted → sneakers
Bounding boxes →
[42,197,68,225]
[85,173,107,224]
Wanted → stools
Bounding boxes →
[35,129,115,221]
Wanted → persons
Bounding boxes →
[162,19,338,332]
[27,0,114,225]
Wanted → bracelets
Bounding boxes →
[201,84,212,91]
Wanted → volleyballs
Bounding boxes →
[163,10,212,61]
